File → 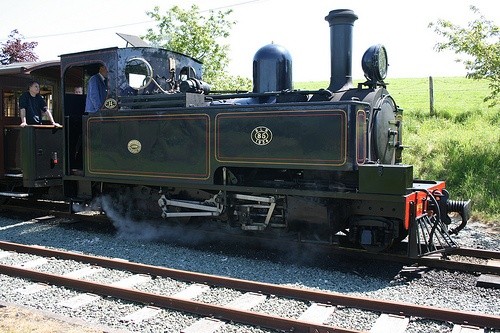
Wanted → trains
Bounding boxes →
[1,8,473,261]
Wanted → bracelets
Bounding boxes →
[51,120,55,122]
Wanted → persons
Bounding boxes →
[74,84,84,94]
[85,64,108,115]
[19,80,60,128]
